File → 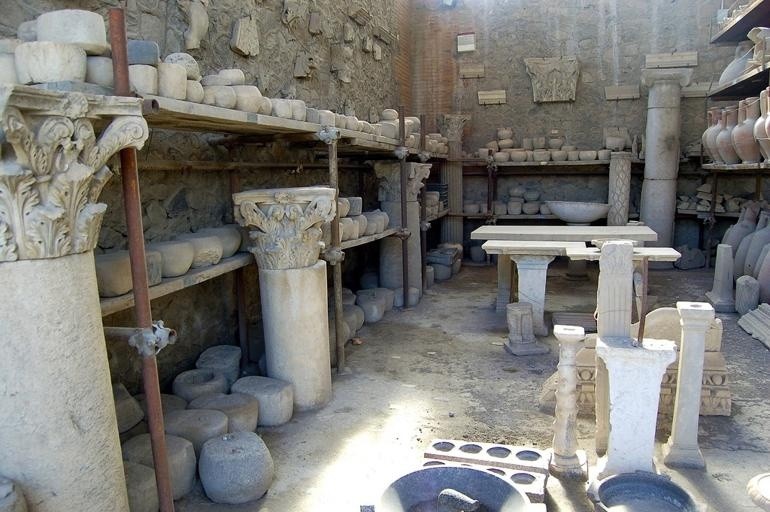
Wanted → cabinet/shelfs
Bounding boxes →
[694,0,770,175]
[11,87,449,318]
[463,159,757,221]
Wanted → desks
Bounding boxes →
[471,225,680,342]
